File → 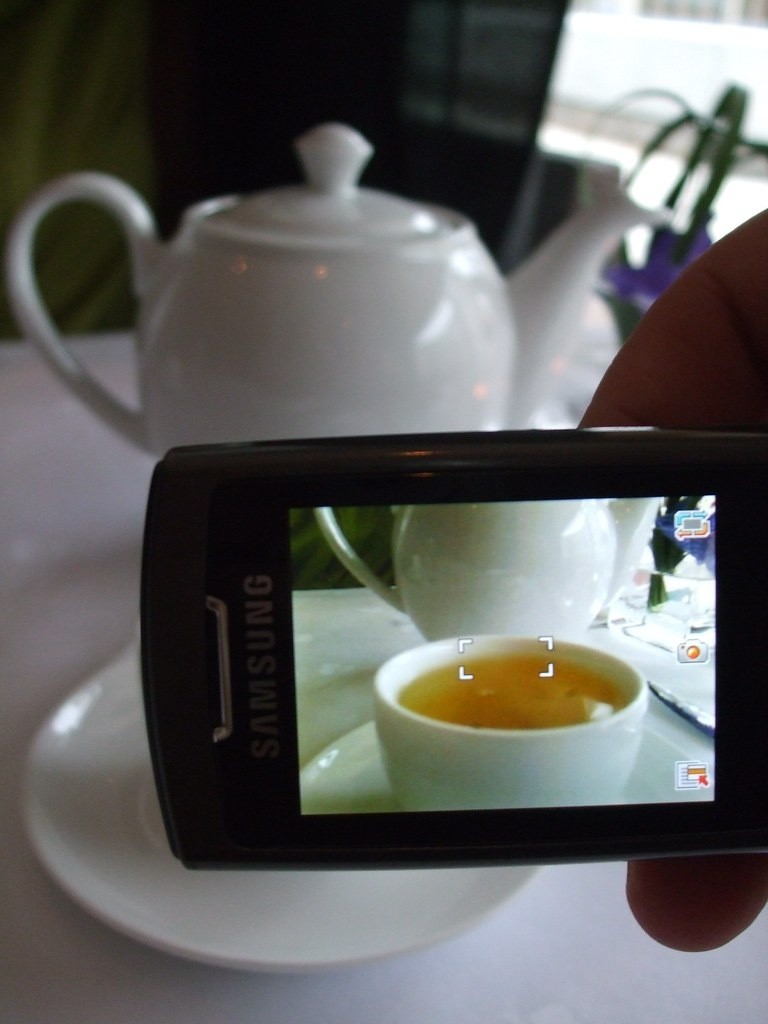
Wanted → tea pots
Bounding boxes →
[311,496,659,644]
[6,122,672,460]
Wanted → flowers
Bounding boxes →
[645,491,715,608]
[597,83,768,341]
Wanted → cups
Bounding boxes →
[373,634,648,806]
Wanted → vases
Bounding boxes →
[620,566,713,650]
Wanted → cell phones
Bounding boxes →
[138,423,768,870]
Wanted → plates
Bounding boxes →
[20,642,542,973]
[300,719,715,814]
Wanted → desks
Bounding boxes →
[0,327,768,1023]
